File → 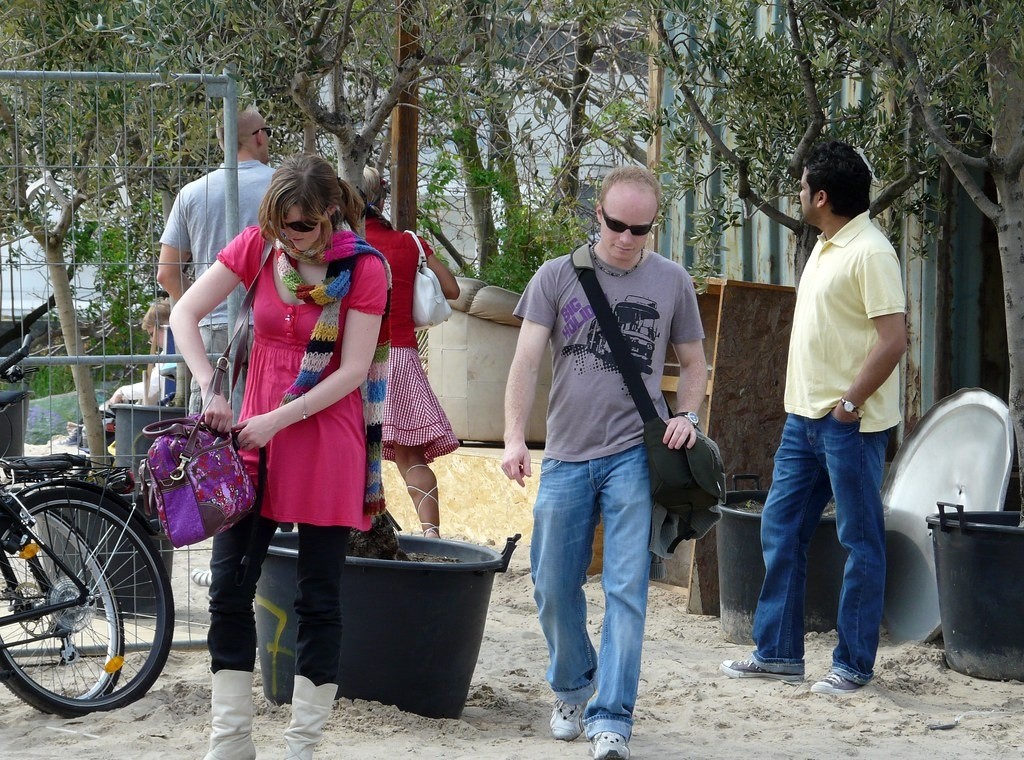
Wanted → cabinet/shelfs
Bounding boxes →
[659,277,798,617]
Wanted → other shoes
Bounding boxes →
[191,571,211,587]
[58,434,77,445]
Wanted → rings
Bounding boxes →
[242,441,248,446]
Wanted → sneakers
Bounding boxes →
[720,659,805,681]
[588,732,630,760]
[550,697,590,741]
[811,672,862,694]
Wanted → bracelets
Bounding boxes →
[302,392,307,419]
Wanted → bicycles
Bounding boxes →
[1,333,179,717]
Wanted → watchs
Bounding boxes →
[841,399,859,413]
[675,412,699,427]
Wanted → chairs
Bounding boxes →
[104,327,175,433]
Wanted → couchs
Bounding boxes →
[427,277,552,447]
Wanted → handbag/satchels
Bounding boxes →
[406,230,453,328]
[139,418,257,548]
[644,417,725,514]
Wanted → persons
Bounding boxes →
[168,152,387,760]
[500,165,708,760]
[47,303,171,448]
[357,165,461,538]
[156,103,280,584]
[720,139,908,695]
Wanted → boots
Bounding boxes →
[283,675,340,760]
[202,667,256,760]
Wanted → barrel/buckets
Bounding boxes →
[927,502,1024,682]
[109,405,188,478]
[255,523,520,718]
[39,486,174,616]
[714,474,848,647]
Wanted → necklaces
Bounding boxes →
[591,241,644,277]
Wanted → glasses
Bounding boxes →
[602,205,655,235]
[252,128,271,137]
[281,220,318,232]
